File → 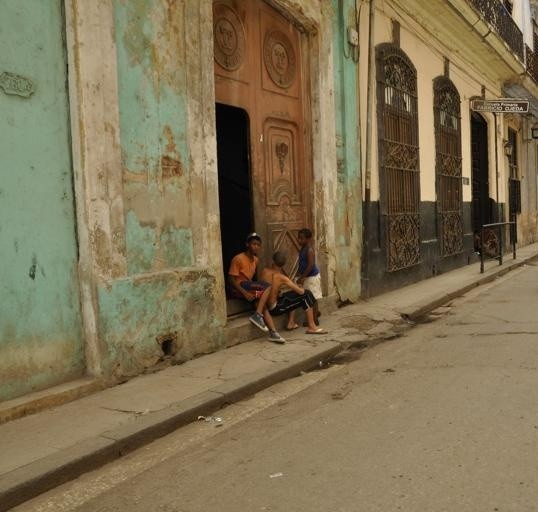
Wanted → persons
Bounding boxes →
[227,228,329,344]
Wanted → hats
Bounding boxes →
[247,232,262,243]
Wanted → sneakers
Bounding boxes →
[286,322,300,331]
[266,330,286,343]
[248,311,270,332]
[304,327,330,334]
